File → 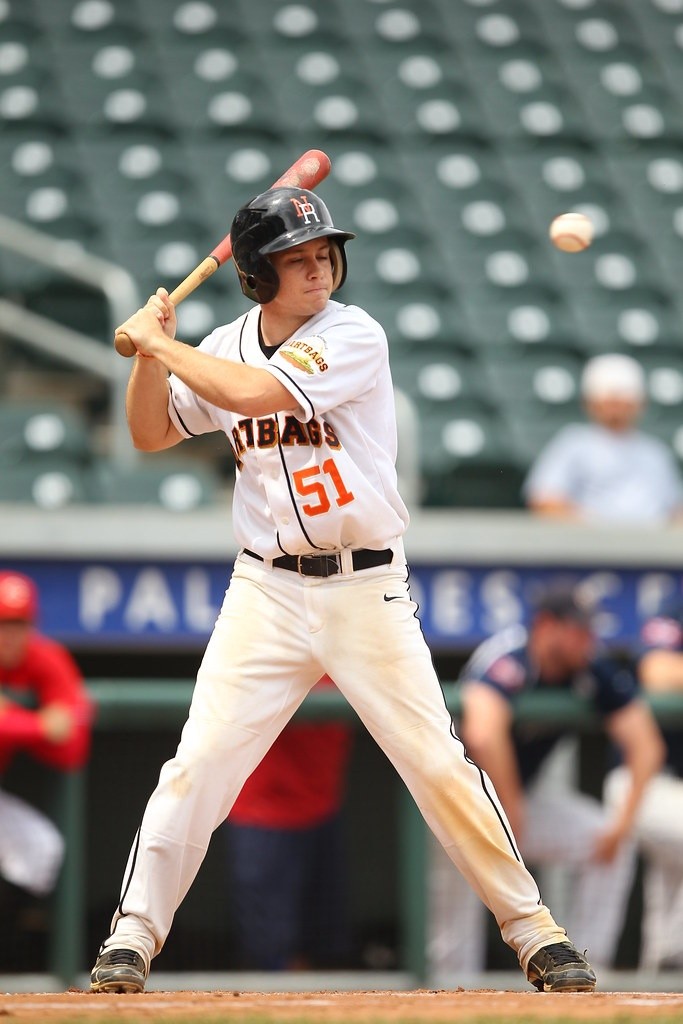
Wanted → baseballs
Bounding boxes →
[549,212,594,251]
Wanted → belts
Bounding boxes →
[242,549,393,579]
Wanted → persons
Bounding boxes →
[524,352,678,521]
[228,674,365,973]
[89,188,599,991]
[0,571,95,967]
[430,584,683,975]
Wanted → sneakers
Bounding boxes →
[90,949,144,993]
[527,941,596,993]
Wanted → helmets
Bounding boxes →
[228,187,356,306]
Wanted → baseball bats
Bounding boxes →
[114,147,332,358]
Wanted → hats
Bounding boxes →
[0,571,36,622]
[583,355,645,402]
